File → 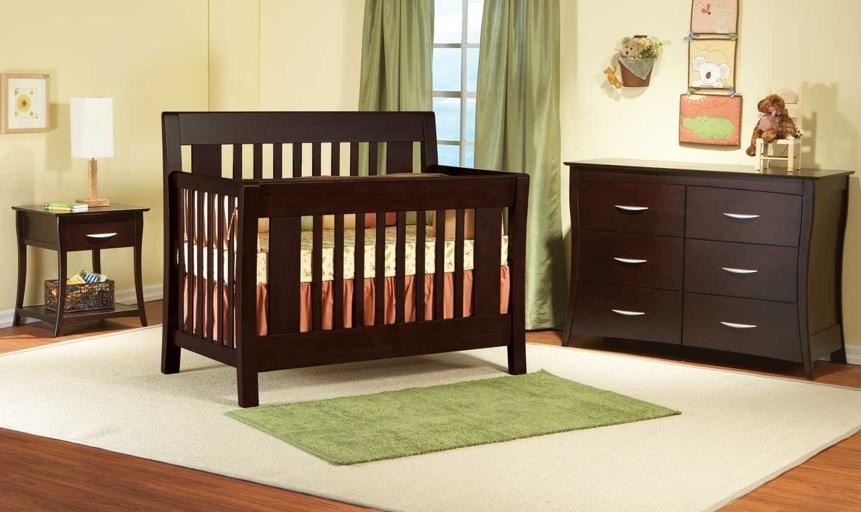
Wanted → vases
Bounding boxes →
[619,58,655,87]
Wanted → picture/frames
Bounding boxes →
[689,0,739,36]
[0,72,52,135]
[686,39,736,93]
[679,93,743,149]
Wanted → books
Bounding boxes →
[42,201,89,215]
[50,270,109,310]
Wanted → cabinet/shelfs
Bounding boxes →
[562,157,687,347]
[682,162,856,382]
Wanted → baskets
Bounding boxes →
[44,280,115,314]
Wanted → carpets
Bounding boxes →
[223,367,683,466]
[1,322,861,512]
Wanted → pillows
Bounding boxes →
[434,209,505,242]
[185,189,262,253]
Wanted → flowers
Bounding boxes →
[635,37,664,58]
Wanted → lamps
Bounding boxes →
[70,97,115,207]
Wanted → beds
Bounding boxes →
[161,110,532,409]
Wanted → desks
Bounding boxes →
[11,203,151,339]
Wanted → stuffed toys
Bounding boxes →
[743,93,800,157]
[602,67,623,89]
[611,36,645,69]
